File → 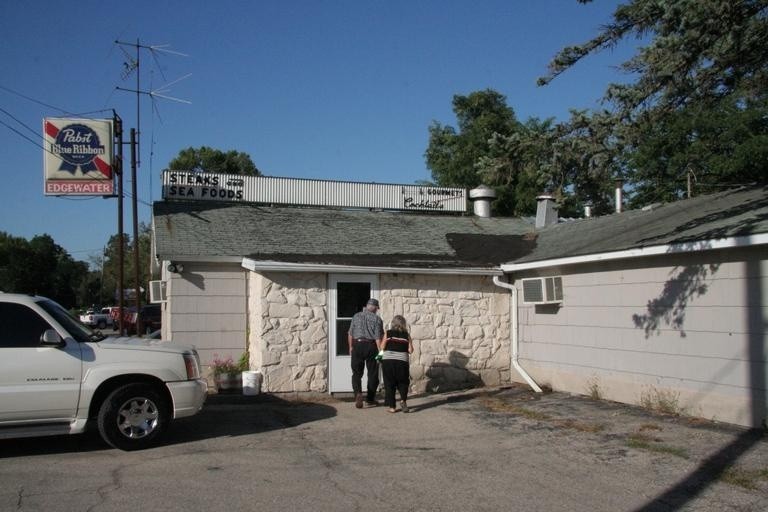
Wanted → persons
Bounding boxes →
[373,314,415,413]
[347,298,387,410]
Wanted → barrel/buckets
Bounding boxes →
[242,371,261,396]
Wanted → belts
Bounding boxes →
[353,338,375,343]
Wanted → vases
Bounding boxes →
[220,373,241,389]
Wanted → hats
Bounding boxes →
[367,298,380,310]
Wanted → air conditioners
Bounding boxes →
[149,280,168,304]
[521,276,563,305]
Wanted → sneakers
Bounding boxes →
[355,393,410,414]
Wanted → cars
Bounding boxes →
[1,293,207,451]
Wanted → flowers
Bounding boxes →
[206,351,250,386]
